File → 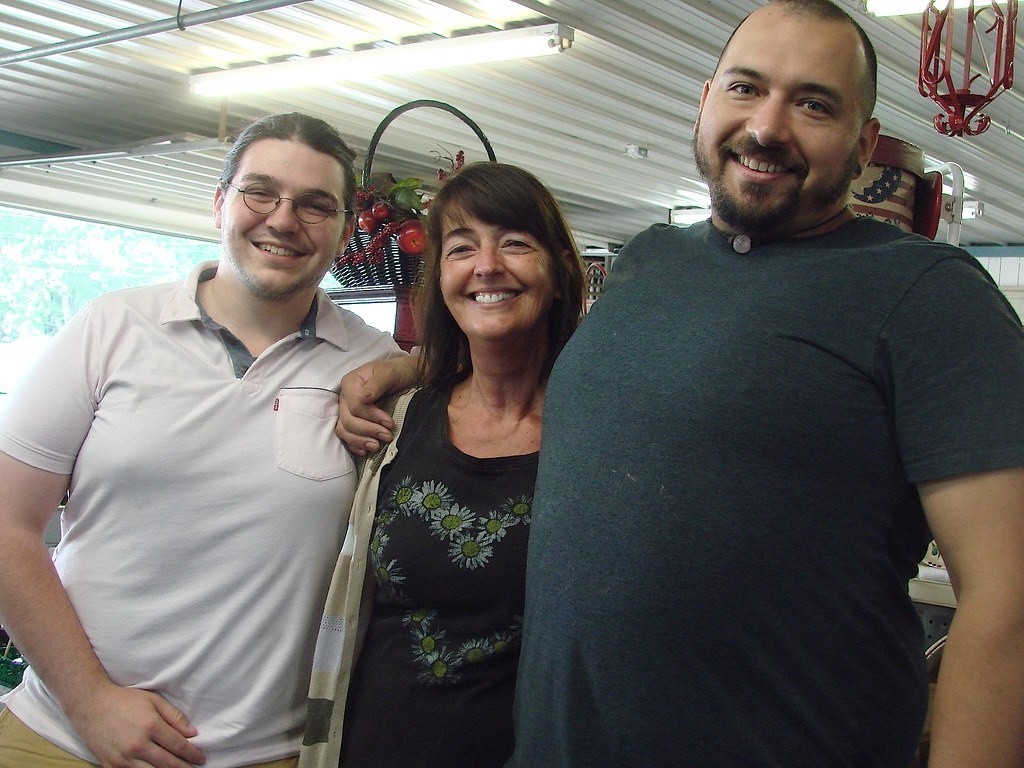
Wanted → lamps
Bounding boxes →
[189,23,574,97]
[669,201,985,224]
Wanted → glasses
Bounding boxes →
[221,180,351,224]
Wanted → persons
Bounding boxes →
[0,113,407,768]
[295,161,593,768]
[337,0,1024,768]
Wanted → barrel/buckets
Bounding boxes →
[848,135,923,234]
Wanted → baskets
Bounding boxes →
[327,99,498,288]
